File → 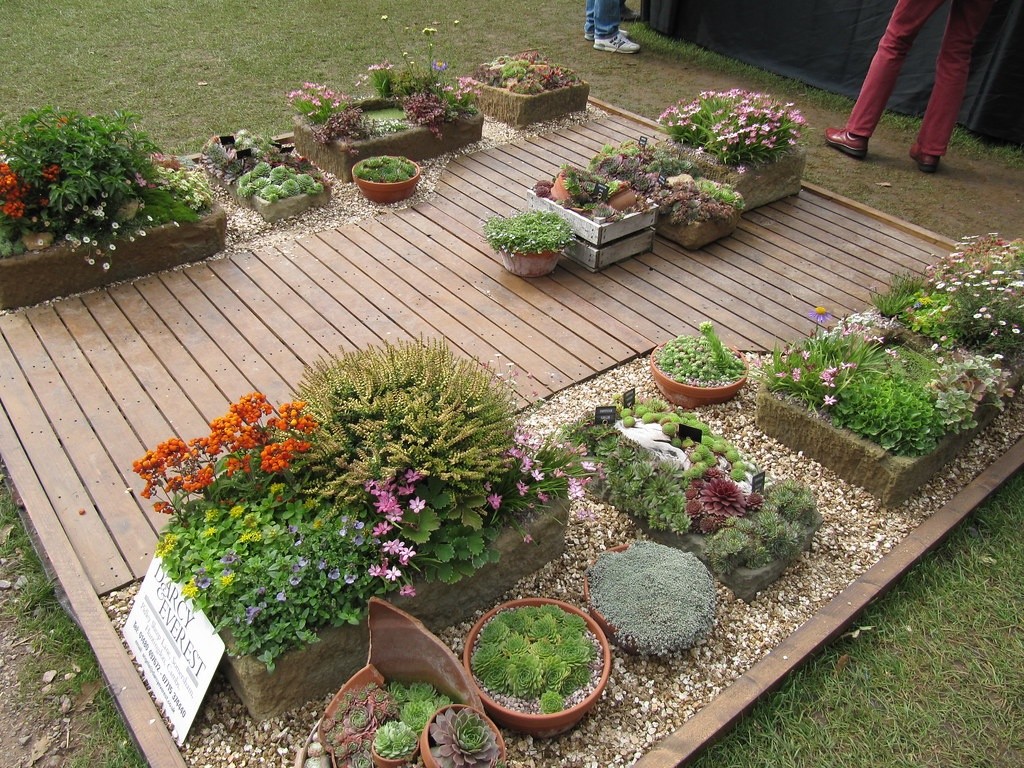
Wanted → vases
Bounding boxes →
[293,94,485,182]
[656,141,806,213]
[2,199,226,312]
[496,245,564,278]
[757,305,1024,507]
[181,499,571,720]
[468,79,590,130]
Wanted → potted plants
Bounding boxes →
[586,143,743,251]
[348,154,420,202]
[461,597,611,736]
[578,542,717,656]
[371,721,420,768]
[527,166,660,273]
[199,132,331,220]
[649,321,748,401]
[552,396,827,600]
[420,705,509,768]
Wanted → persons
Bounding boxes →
[584,0,643,53]
[825,0,992,173]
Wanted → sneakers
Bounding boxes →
[592,32,640,54]
[584,27,630,41]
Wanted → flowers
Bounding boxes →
[657,88,809,161]
[478,48,582,94]
[0,109,210,272]
[130,339,580,659]
[478,209,577,257]
[286,12,480,136]
[750,234,1024,458]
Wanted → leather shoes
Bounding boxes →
[909,143,940,173]
[824,127,869,158]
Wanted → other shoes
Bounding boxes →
[619,7,640,22]
